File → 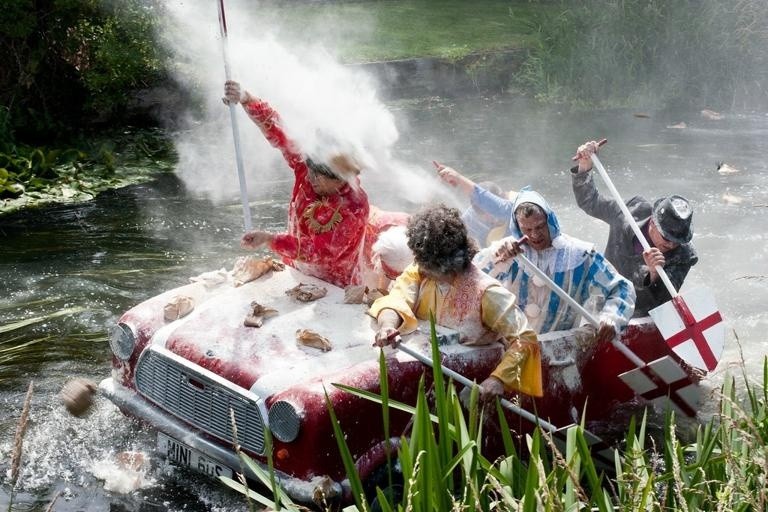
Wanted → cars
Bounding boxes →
[98,209,681,510]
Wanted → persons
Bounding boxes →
[363,204,545,406]
[470,191,637,344]
[436,161,521,248]
[221,79,370,290]
[341,206,416,308]
[569,140,698,320]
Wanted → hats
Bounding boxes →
[312,151,364,189]
[652,195,694,243]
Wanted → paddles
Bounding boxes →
[215,0,252,234]
[495,235,703,420]
[572,139,725,370]
[373,330,620,474]
